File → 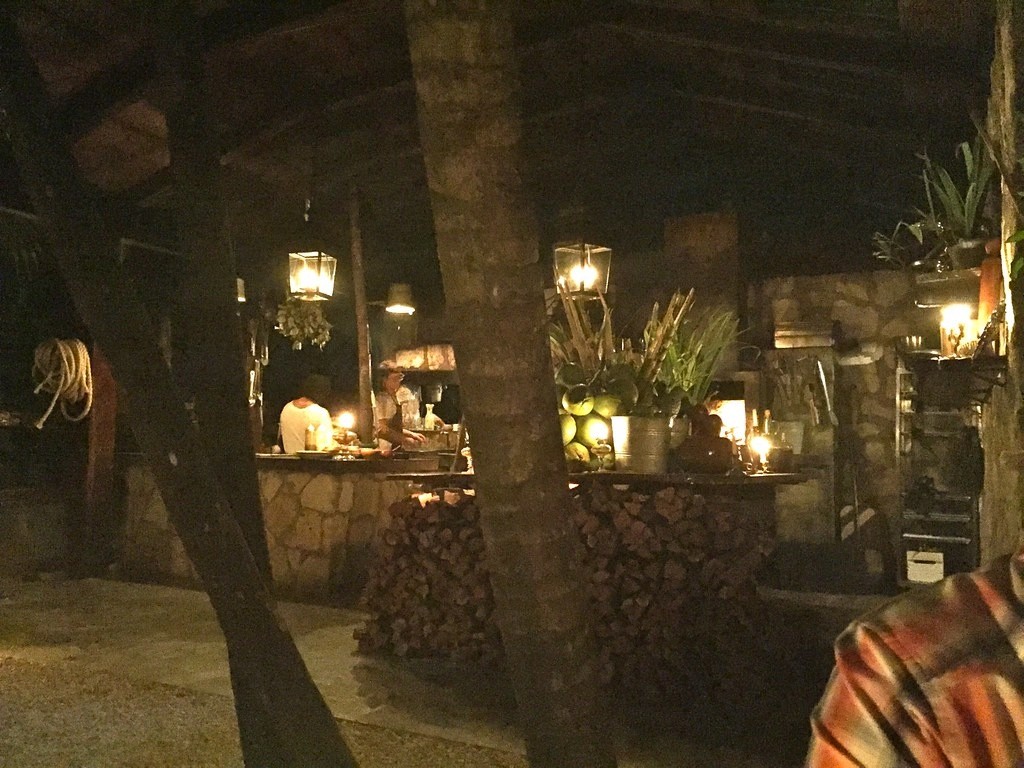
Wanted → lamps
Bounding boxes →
[551,220,615,302]
[385,282,416,315]
[285,236,339,302]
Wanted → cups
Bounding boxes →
[304,427,316,450]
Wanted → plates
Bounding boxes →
[295,449,331,454]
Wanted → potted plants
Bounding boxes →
[912,136,996,270]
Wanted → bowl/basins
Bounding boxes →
[890,332,926,350]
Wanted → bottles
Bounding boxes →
[775,431,794,471]
[425,404,435,429]
